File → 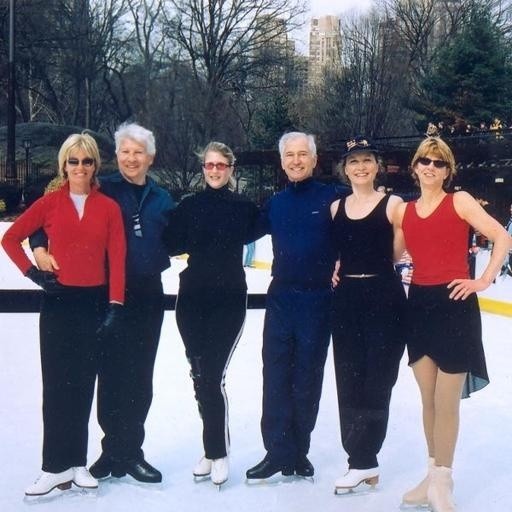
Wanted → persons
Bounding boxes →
[330,140,404,489]
[30,123,179,482]
[330,137,512,512]
[374,156,512,286]
[427,116,504,138]
[244,130,386,479]
[243,239,256,268]
[170,142,260,484]
[1,133,128,496]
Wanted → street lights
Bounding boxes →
[22,133,32,178]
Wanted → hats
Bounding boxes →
[342,136,379,156]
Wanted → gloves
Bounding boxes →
[97,301,125,347]
[23,266,68,296]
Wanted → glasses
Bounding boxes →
[203,162,233,169]
[283,151,314,159]
[417,156,448,168]
[66,158,93,166]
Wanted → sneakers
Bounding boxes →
[24,444,163,496]
[245,448,315,479]
[402,456,455,512]
[336,464,381,490]
[191,454,231,484]
